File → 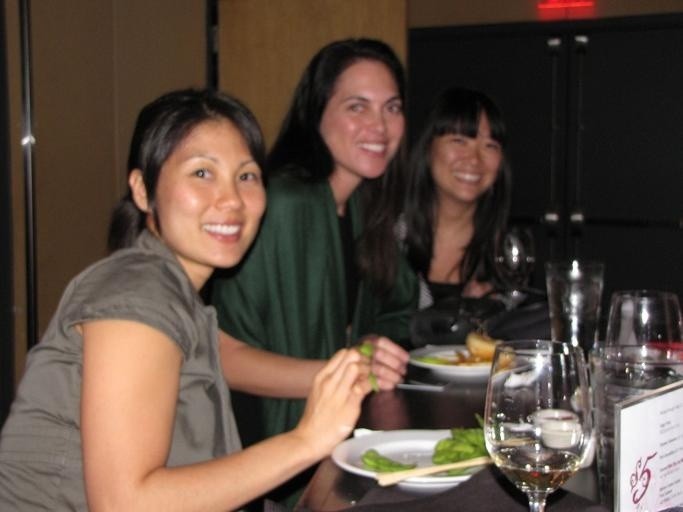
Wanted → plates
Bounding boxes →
[330,430,495,488]
[408,343,539,377]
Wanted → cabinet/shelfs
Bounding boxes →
[406,12,683,337]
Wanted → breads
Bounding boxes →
[466,331,517,372]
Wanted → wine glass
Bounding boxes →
[483,340,597,512]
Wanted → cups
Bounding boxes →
[587,340,683,512]
[545,261,605,352]
[607,289,683,345]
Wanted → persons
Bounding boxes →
[389,83,520,343]
[200,38,419,512]
[0,85,377,511]
